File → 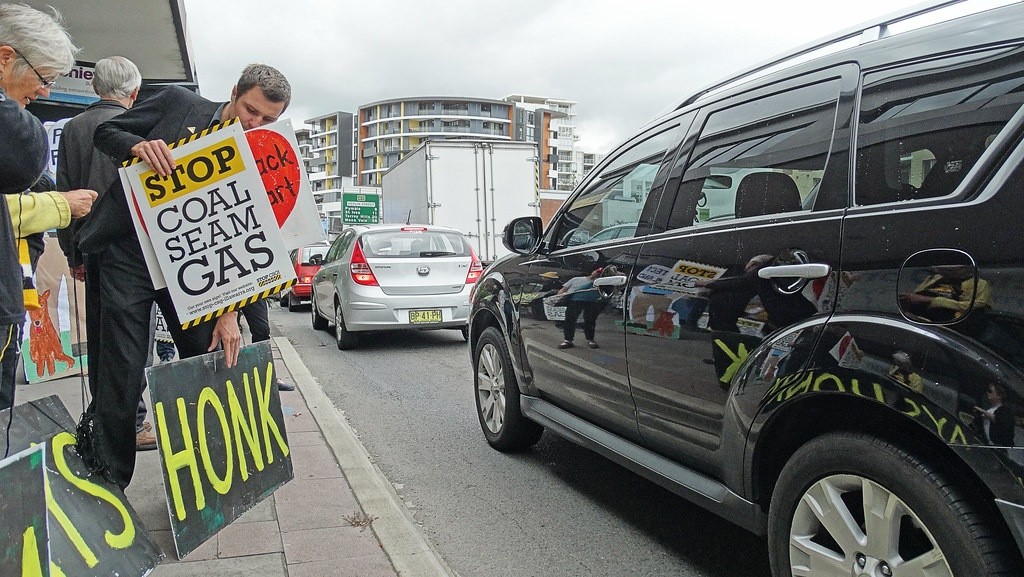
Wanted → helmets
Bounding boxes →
[894,351,912,370]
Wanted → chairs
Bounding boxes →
[734,172,803,220]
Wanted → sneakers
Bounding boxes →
[587,340,600,349]
[276,377,295,391]
[558,339,574,349]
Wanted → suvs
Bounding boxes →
[470,0,1024,577]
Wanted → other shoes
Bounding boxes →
[136,422,157,451]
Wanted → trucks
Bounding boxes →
[381,140,542,270]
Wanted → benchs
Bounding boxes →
[913,146,984,200]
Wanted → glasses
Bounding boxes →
[987,389,998,396]
[14,50,58,92]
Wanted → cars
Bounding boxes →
[584,223,636,243]
[309,223,485,350]
[267,240,333,312]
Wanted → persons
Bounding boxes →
[890,352,922,393]
[237,300,295,391]
[531,271,562,321]
[56,55,158,452]
[77,64,291,493]
[901,256,994,333]
[557,267,607,349]
[0,1,98,412]
[968,383,1014,457]
[697,253,828,390]
